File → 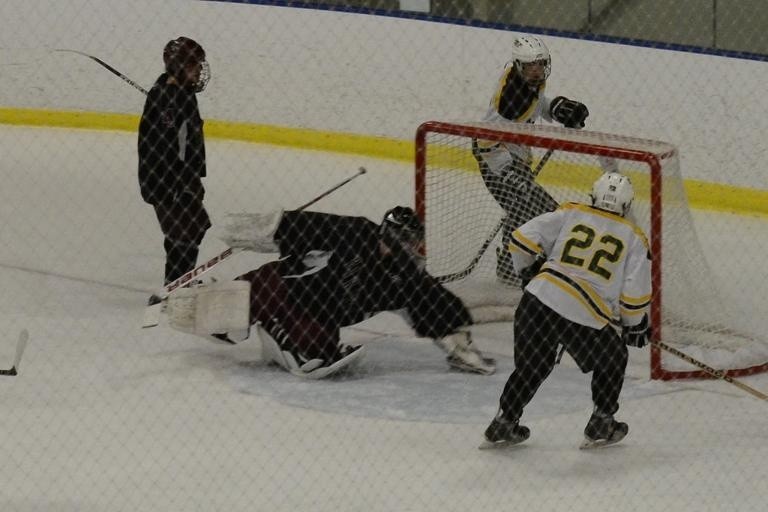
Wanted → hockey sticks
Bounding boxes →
[142,166,366,328]
[1,330,29,377]
[432,124,574,283]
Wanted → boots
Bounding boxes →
[485,402,530,443]
[585,404,628,441]
[495,249,521,283]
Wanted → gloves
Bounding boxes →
[621,312,652,347]
[522,257,542,289]
[499,165,529,196]
[550,97,590,128]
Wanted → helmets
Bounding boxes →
[163,36,210,94]
[590,171,635,217]
[512,35,552,86]
[377,206,425,260]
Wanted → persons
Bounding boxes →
[138,38,211,285]
[485,173,657,446]
[164,204,495,377]
[472,36,588,283]
